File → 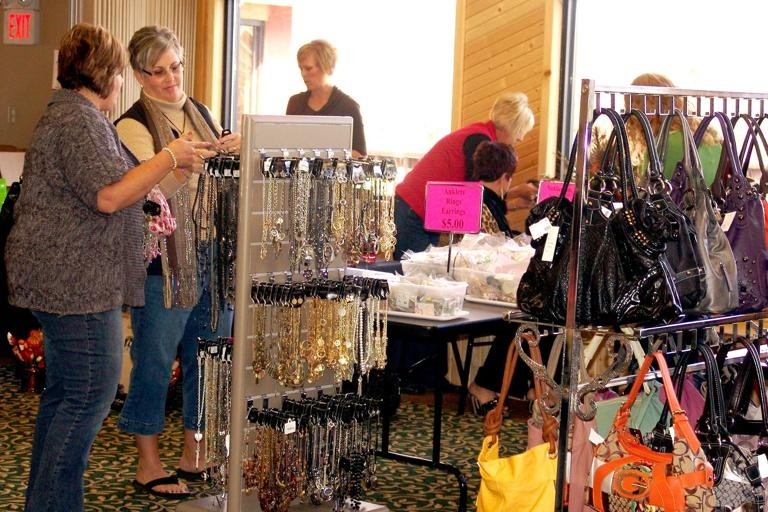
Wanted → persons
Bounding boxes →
[3,22,215,512]
[440,142,555,421]
[395,91,537,394]
[112,24,242,498]
[285,39,367,161]
[627,76,720,391]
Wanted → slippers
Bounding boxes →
[175,466,217,479]
[134,477,193,500]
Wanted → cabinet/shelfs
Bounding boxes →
[505,79,768,512]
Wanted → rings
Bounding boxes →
[199,154,206,160]
[233,147,237,151]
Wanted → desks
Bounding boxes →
[361,298,530,512]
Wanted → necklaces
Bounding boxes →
[191,172,398,512]
[162,110,187,138]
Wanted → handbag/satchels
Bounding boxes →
[516,109,767,328]
[0,181,21,306]
[477,333,767,511]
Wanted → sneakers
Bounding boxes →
[427,377,455,394]
[401,381,425,395]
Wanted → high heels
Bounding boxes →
[468,392,510,419]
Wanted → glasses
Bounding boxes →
[142,62,185,77]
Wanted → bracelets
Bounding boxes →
[162,147,177,170]
